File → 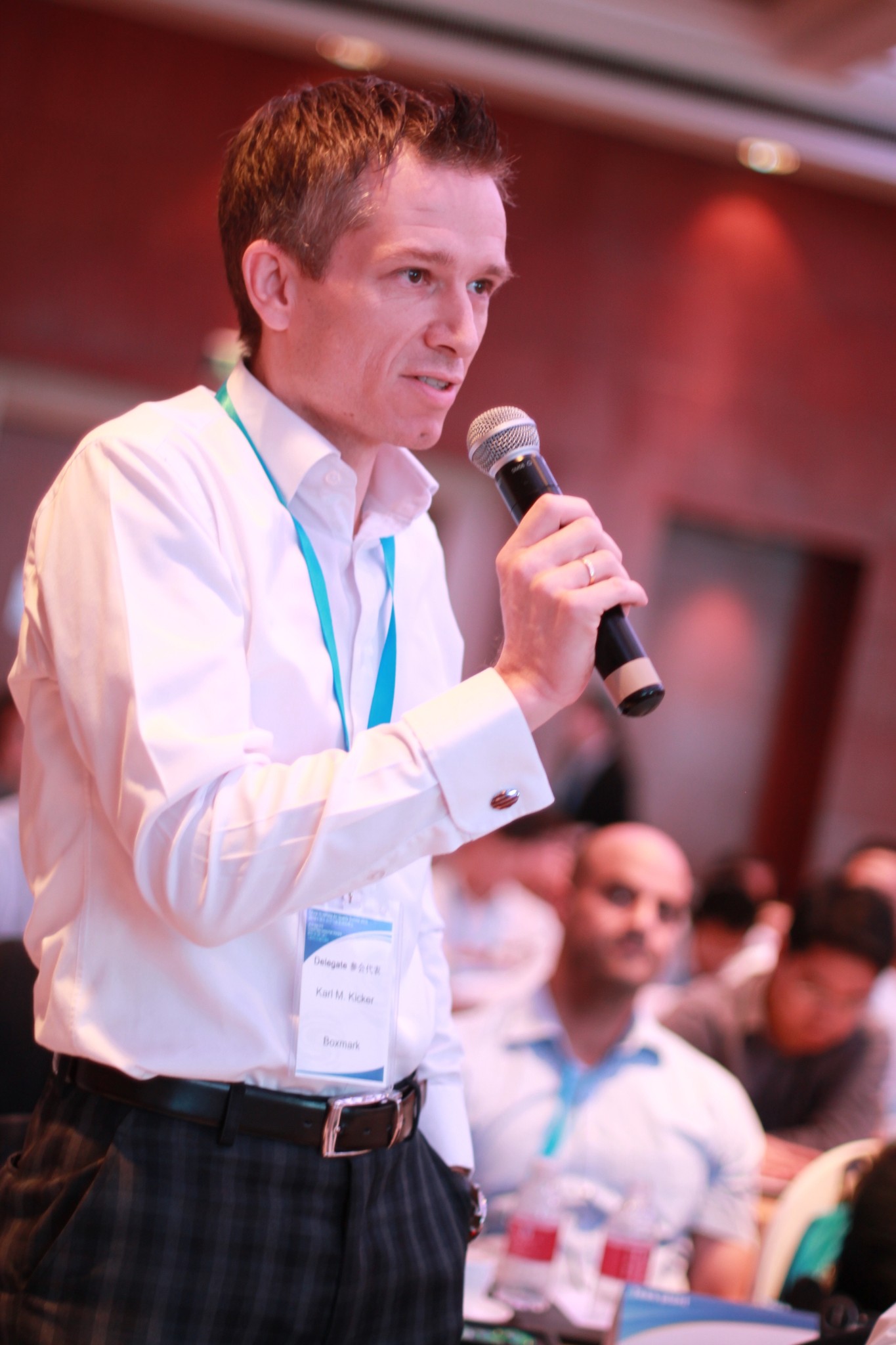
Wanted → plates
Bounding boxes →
[463,1287,514,1326]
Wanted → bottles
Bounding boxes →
[587,1193,655,1325]
[493,1153,563,1310]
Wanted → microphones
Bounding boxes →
[467,406,666,718]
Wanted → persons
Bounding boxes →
[0,688,37,945]
[428,685,896,1345]
[0,78,647,1345]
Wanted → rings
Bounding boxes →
[579,557,594,586]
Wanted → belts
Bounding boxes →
[57,1054,429,1159]
